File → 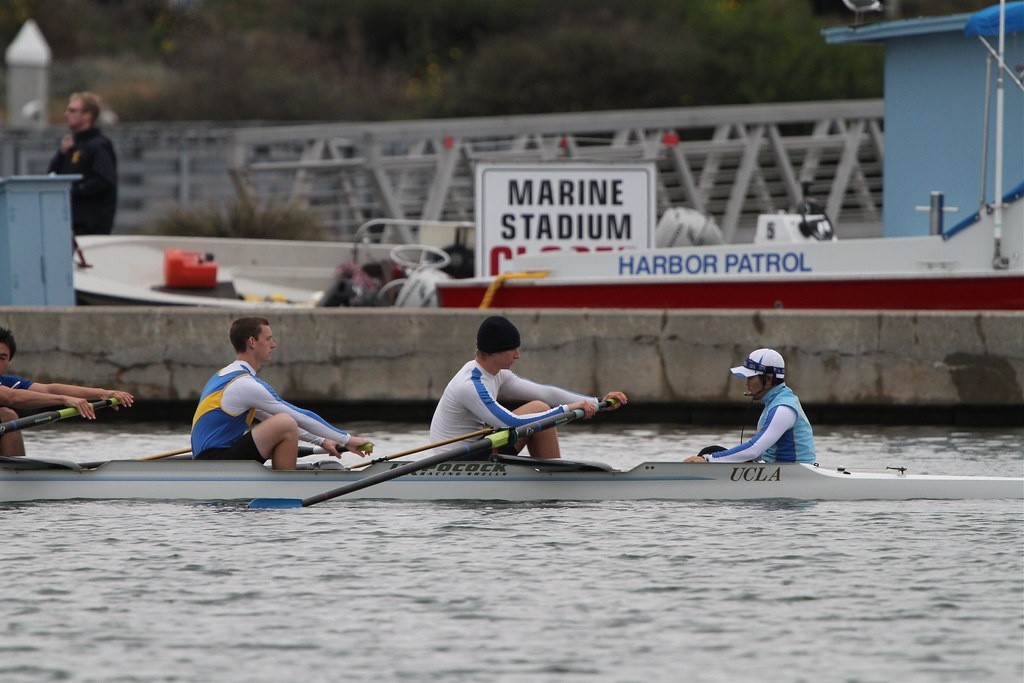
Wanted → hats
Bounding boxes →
[476,316,520,354]
[730,348,786,381]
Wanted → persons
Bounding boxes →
[683,348,816,466]
[191,317,373,471]
[48,92,117,235]
[429,315,627,462]
[0,327,134,457]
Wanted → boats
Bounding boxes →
[74,0,1023,311]
[0,453,1024,501]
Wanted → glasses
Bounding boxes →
[68,107,89,113]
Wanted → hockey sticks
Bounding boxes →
[0,397,123,436]
[247,397,618,510]
[297,443,373,458]
